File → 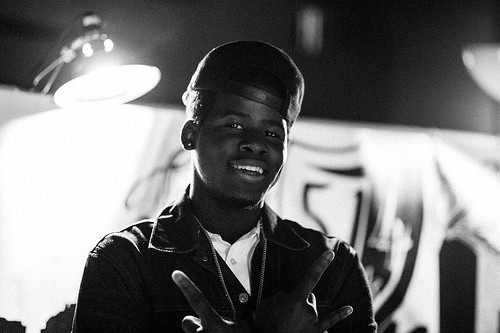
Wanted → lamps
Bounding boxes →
[33,13,162,108]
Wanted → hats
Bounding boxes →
[182,40,306,129]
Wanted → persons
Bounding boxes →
[72,39,378,333]
